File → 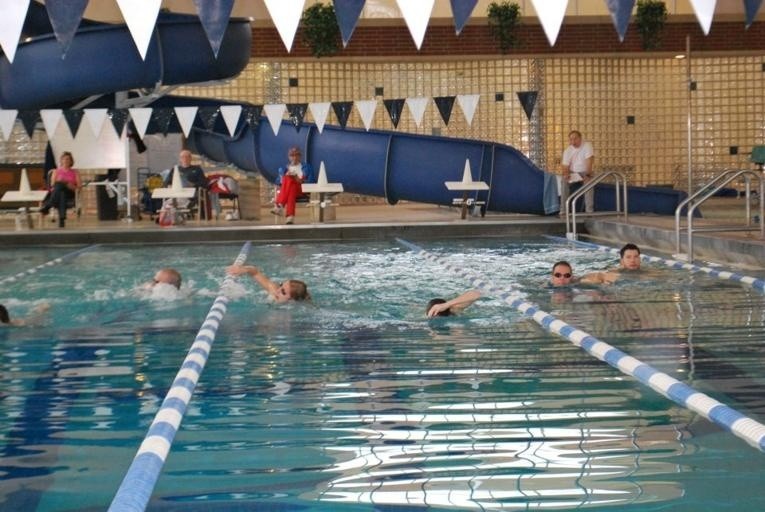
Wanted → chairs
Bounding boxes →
[45,169,83,222]
[167,187,208,227]
[274,181,315,224]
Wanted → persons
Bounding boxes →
[270,144,316,224]
[608,243,655,284]
[556,131,596,219]
[150,268,181,292]
[0,301,51,328]
[163,149,205,188]
[38,151,82,227]
[540,262,613,291]
[426,289,481,318]
[226,265,313,304]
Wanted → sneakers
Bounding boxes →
[286,217,292,224]
[59,218,64,227]
[271,207,282,215]
[39,208,48,214]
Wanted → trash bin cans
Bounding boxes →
[95,174,118,220]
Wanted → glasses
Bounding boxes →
[279,282,286,295]
[554,272,570,278]
